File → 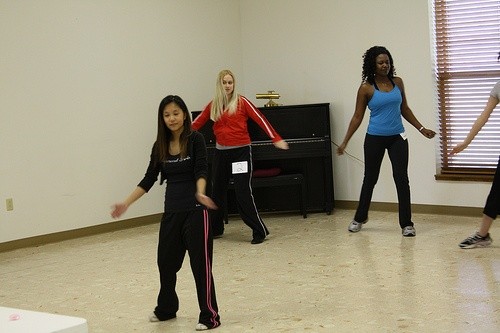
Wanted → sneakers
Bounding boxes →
[348,218,368,232]
[458,232,492,249]
[402,226,416,236]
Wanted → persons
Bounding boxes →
[338,47,436,237]
[448,51,500,249]
[112,95,222,331]
[191,70,289,245]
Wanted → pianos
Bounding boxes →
[191,102,335,219]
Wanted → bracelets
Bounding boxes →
[419,127,424,131]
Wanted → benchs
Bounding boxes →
[224,174,307,224]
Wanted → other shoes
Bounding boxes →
[251,238,265,244]
[196,323,208,330]
[149,314,159,322]
